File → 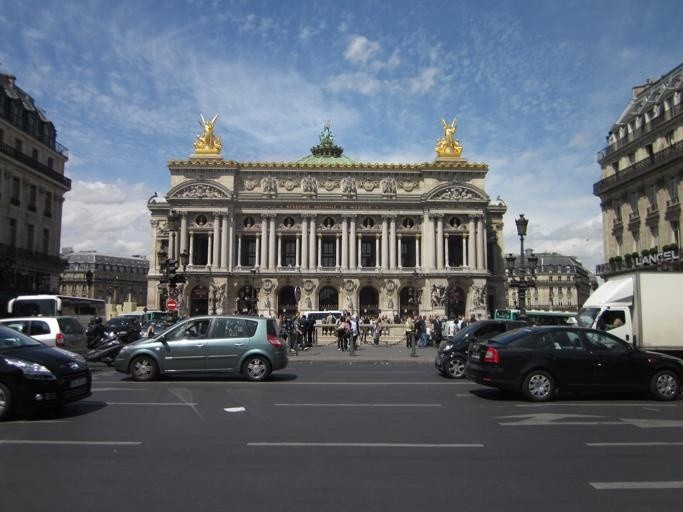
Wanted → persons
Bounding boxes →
[85,305,392,353]
[30,309,43,317]
[395,310,478,347]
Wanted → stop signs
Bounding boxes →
[165,299,177,310]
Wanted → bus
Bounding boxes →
[5,292,108,332]
[495,306,581,330]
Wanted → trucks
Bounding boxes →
[573,267,682,353]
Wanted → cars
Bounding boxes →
[434,317,530,379]
[459,324,682,405]
[0,324,92,421]
[0,311,291,382]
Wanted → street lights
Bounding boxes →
[155,243,192,316]
[249,268,257,315]
[407,284,421,357]
[501,210,539,325]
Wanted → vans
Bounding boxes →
[300,310,343,333]
